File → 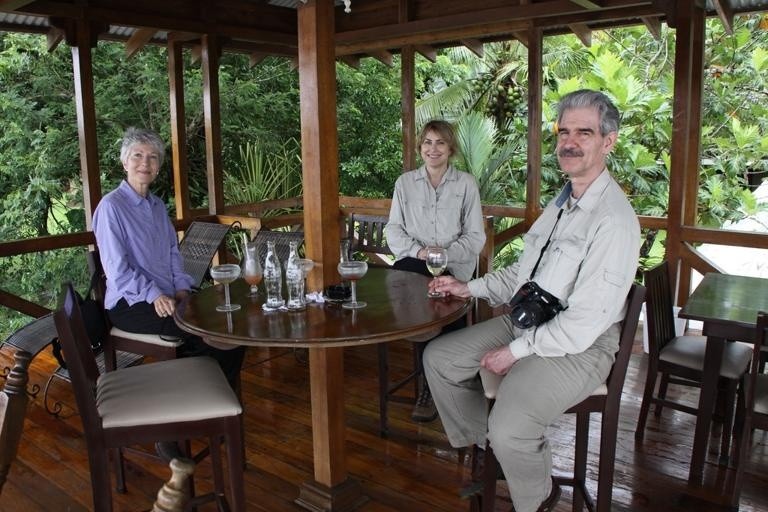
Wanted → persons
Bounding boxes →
[92,130,244,466]
[422,89,640,512]
[383,120,486,422]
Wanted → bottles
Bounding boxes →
[286,241,307,309]
[264,240,283,309]
[339,239,353,297]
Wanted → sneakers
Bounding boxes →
[508,476,561,512]
[412,372,437,423]
[154,442,180,463]
[458,443,497,500]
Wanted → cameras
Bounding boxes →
[508,282,566,330]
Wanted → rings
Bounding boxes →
[161,311,166,316]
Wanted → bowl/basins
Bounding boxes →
[324,285,352,300]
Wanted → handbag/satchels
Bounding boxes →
[75,290,112,356]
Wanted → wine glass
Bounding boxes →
[337,261,369,311]
[284,260,314,305]
[425,247,449,299]
[210,264,243,313]
[240,242,263,298]
